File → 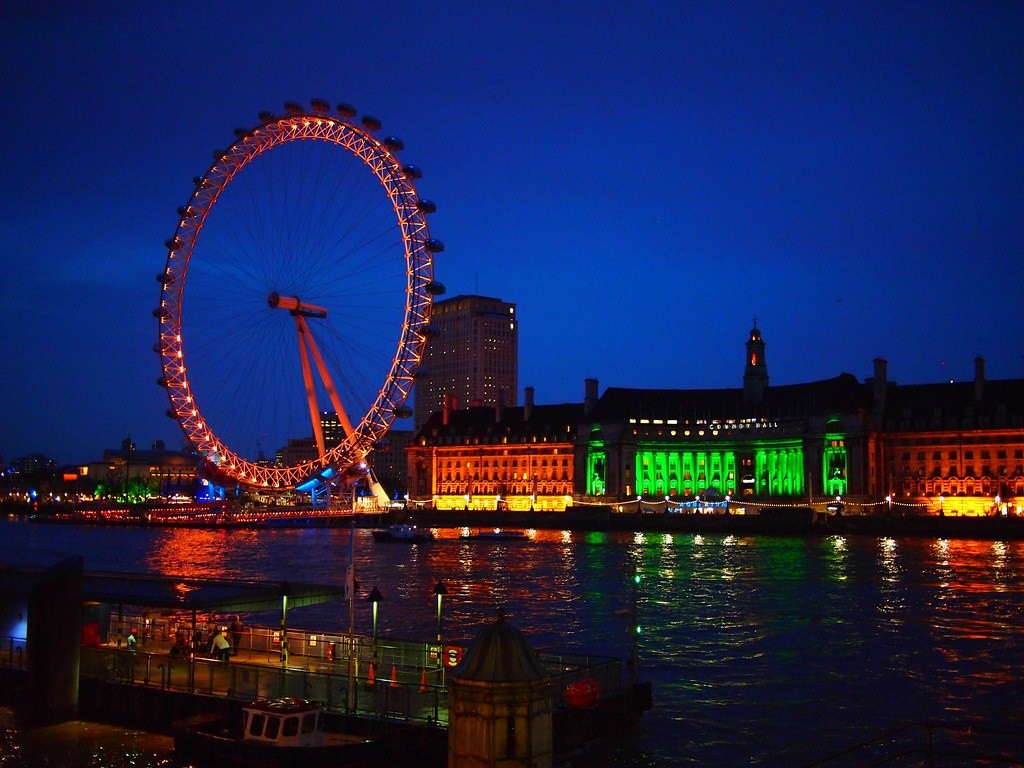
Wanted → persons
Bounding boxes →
[225,616,244,656]
[127,628,138,650]
[168,630,230,663]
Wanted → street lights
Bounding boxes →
[432,578,448,681]
[276,580,292,661]
[366,587,383,680]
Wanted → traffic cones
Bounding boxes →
[419,670,427,694]
[389,666,399,688]
[367,663,375,684]
[326,644,332,661]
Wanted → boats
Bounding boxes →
[187,696,374,768]
[370,525,435,544]
[0,565,655,755]
[460,531,530,541]
